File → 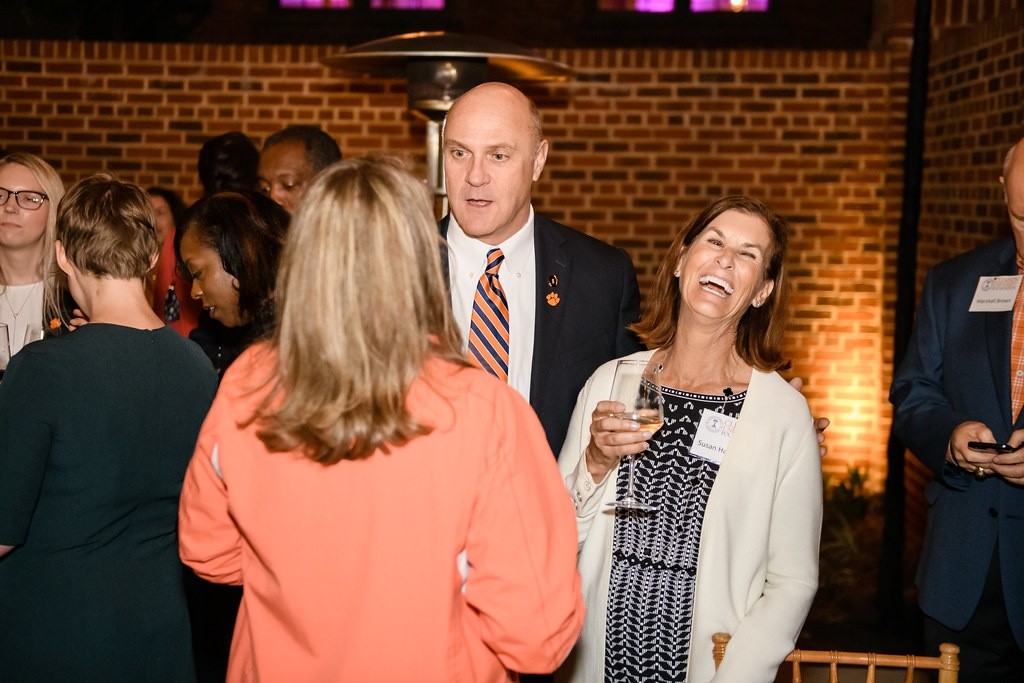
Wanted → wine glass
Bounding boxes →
[606,360,658,510]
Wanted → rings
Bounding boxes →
[976,466,984,476]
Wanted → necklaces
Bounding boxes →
[4,280,38,356]
[631,347,741,559]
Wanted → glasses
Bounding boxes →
[0,186,48,210]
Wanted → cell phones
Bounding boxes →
[967,441,1016,454]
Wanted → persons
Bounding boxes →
[145,188,202,340]
[173,186,294,375]
[0,150,219,683]
[889,131,1024,683]
[556,194,824,683]
[260,124,340,215]
[198,132,260,196]
[434,83,648,459]
[178,152,588,683]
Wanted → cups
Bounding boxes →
[0,322,11,383]
[23,323,62,348]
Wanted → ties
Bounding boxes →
[467,248,510,384]
